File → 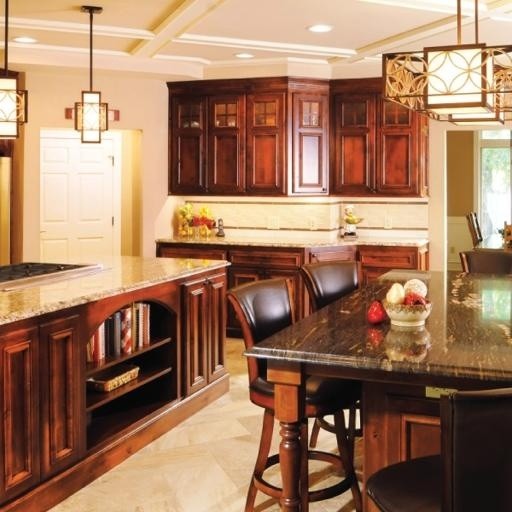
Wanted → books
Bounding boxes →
[86,302,150,362]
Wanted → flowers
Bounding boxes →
[496,221,512,242]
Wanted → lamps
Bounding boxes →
[1,0,31,142]
[381,0,512,127]
[73,6,109,144]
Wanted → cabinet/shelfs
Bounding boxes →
[177,267,230,398]
[284,76,331,197]
[355,244,430,286]
[89,280,185,454]
[1,306,89,506]
[303,245,359,318]
[166,80,209,196]
[209,79,284,198]
[156,243,230,338]
[230,246,304,338]
[328,78,429,198]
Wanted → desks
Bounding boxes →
[243,267,510,510]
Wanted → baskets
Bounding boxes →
[86,364,140,393]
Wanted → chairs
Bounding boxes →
[470,212,484,241]
[364,384,511,511]
[226,277,364,511]
[458,250,512,273]
[463,213,480,247]
[298,261,361,450]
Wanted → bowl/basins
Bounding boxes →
[381,329,434,354]
[382,296,434,327]
